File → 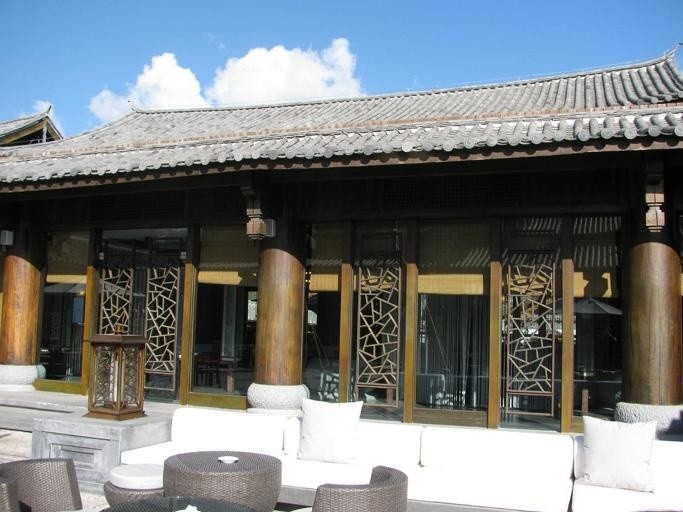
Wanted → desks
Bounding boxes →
[163,450,282,511]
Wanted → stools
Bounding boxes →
[103,463,163,507]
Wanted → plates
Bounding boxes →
[217,456,239,464]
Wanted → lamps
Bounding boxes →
[85,322,146,420]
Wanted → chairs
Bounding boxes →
[0,457,82,512]
[311,466,408,511]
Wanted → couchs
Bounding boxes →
[119,405,683,511]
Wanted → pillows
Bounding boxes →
[296,397,363,465]
[574,413,660,497]
